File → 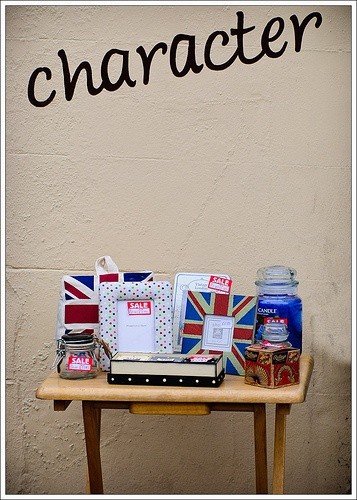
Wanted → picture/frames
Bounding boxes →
[98,280,174,372]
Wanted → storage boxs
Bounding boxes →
[106,350,225,388]
[243,343,302,390]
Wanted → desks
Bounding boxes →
[32,353,318,494]
